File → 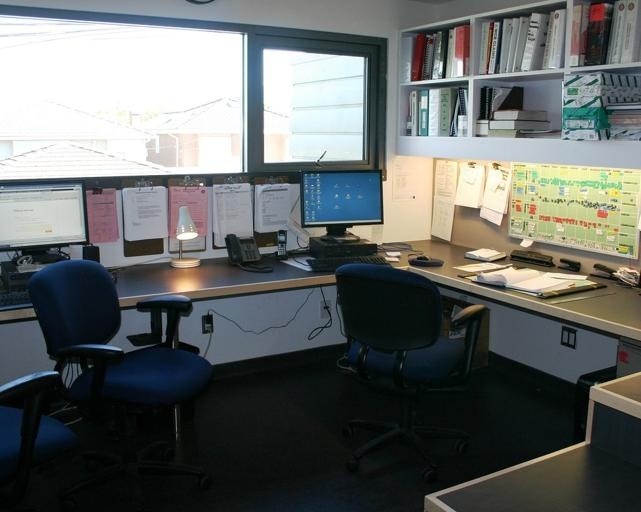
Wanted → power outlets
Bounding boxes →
[320,300,330,320]
[202,314,214,334]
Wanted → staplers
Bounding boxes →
[589,263,618,281]
[558,258,581,272]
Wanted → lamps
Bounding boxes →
[170,205,201,268]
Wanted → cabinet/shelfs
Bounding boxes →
[397,0,640,170]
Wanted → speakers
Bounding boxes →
[83,246,100,263]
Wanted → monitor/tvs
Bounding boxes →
[301,168,384,229]
[0,179,90,251]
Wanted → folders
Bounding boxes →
[420,89,428,136]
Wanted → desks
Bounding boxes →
[1,239,641,393]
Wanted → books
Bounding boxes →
[476,85,551,138]
[464,248,508,262]
[606,102,641,125]
[472,267,573,294]
[406,86,467,136]
[403,24,470,81]
[478,8,565,75]
[569,0,641,67]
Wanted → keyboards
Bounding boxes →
[0,289,33,311]
[307,255,392,273]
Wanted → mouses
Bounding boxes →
[417,255,432,261]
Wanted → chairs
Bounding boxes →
[0,374,119,512]
[333,263,489,482]
[27,260,214,511]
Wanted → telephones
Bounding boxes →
[275,230,289,260]
[225,234,261,264]
